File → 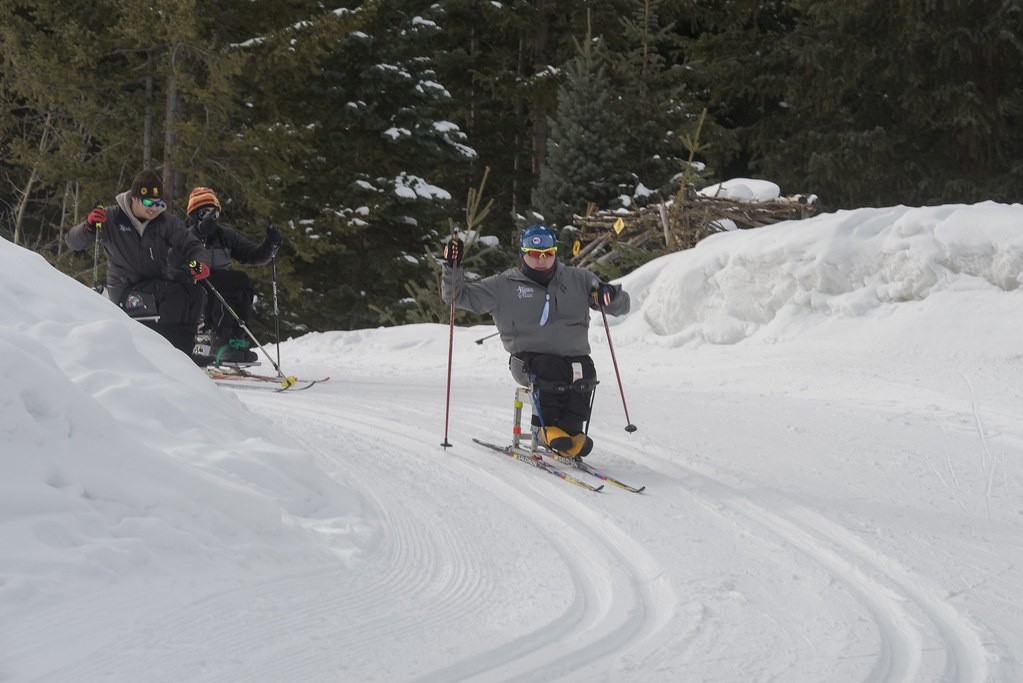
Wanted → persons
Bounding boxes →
[442,226,630,456]
[186,187,283,363]
[65,171,217,366]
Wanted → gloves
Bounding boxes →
[265,225,281,249]
[590,282,616,306]
[446,238,464,268]
[190,262,211,280]
[84,207,107,231]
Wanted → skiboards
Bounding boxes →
[471,437,647,494]
[208,371,330,393]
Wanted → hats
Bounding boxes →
[187,187,222,215]
[131,168,164,198]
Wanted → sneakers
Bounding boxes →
[557,432,593,460]
[529,424,572,451]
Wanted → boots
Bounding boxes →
[208,313,261,367]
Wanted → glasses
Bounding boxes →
[198,208,221,219]
[140,198,161,207]
[523,248,556,258]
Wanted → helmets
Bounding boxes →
[519,224,557,250]
